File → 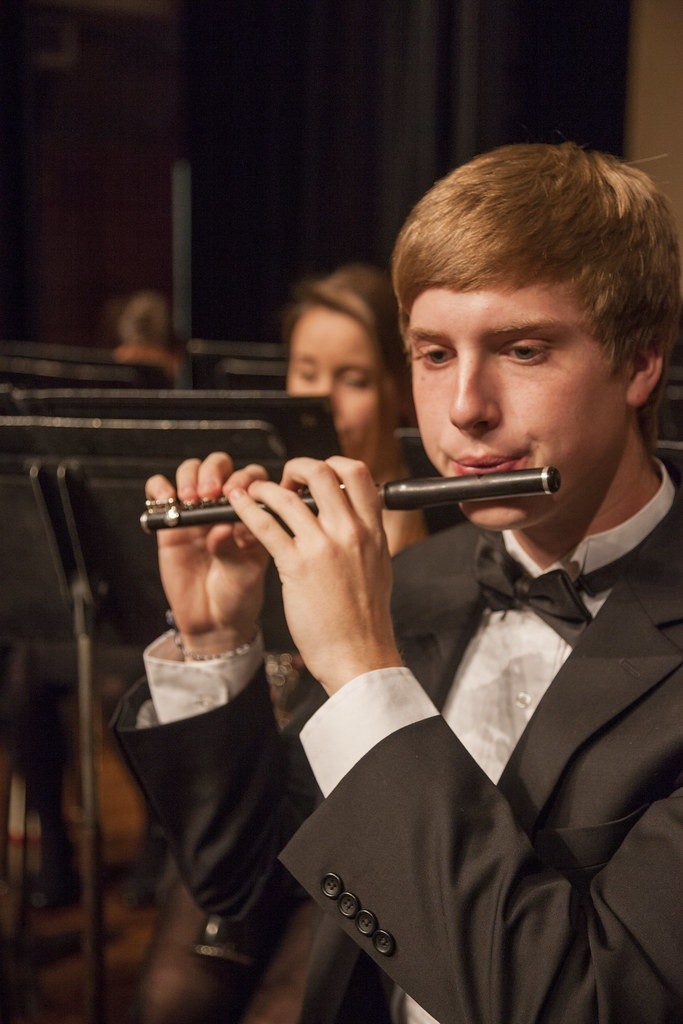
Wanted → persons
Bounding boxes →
[126,252,454,1019]
[106,139,681,1021]
[111,290,192,373]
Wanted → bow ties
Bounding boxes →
[473,528,658,647]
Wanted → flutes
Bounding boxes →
[141,465,562,534]
